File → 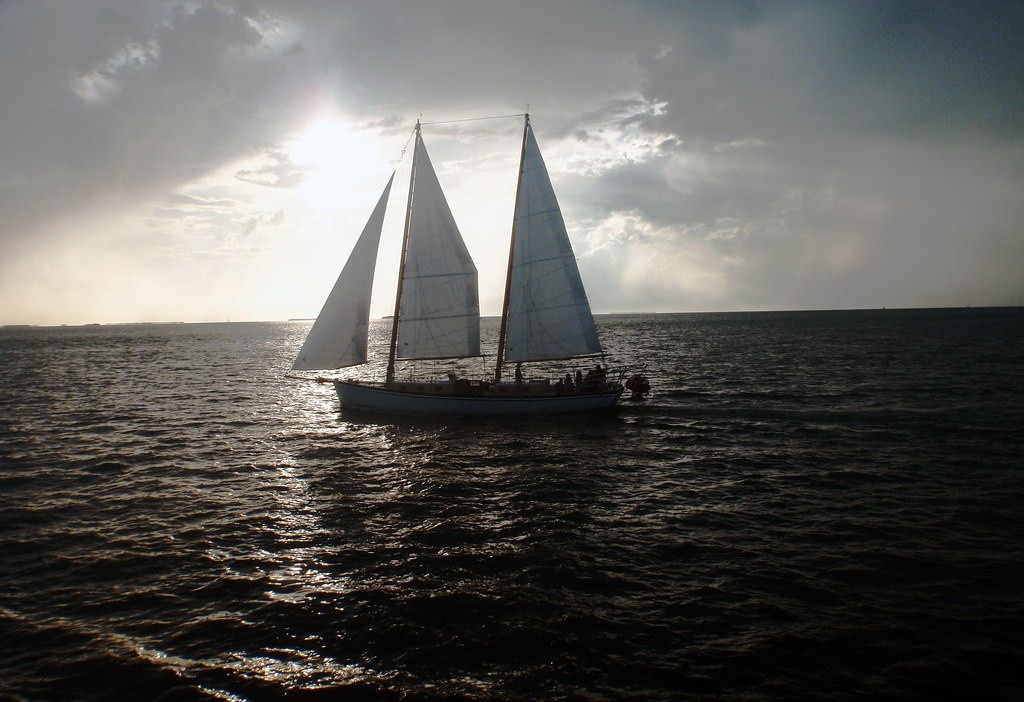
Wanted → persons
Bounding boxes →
[563,372,574,393]
[595,364,602,370]
[553,378,564,394]
[574,370,584,387]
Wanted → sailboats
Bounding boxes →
[284,102,647,417]
